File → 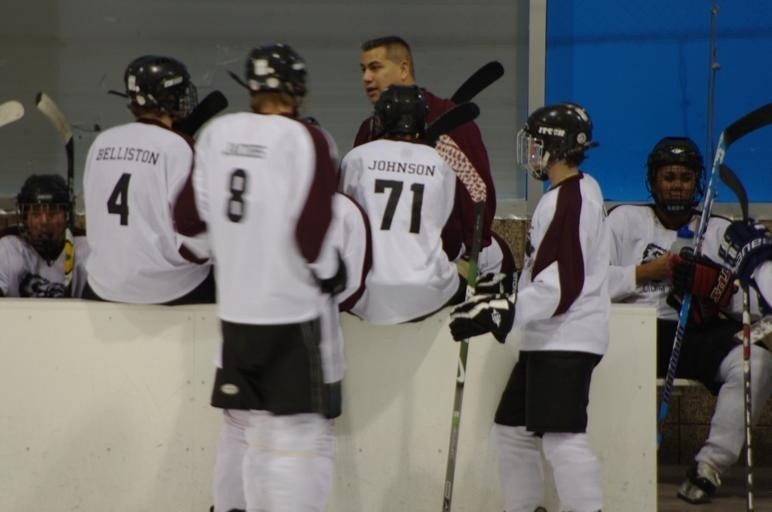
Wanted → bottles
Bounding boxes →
[668,228,695,283]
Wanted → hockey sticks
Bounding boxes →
[717,164,753,511]
[657,102,771,448]
[434,136,487,509]
[35,93,74,300]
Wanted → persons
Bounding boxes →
[475,234,515,294]
[605,136,772,501]
[445,102,613,512]
[0,173,87,297]
[339,84,465,325]
[337,36,495,250]
[189,41,347,511]
[76,54,215,305]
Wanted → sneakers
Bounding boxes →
[674,468,720,505]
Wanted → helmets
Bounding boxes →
[370,84,430,139]
[642,134,710,232]
[122,52,200,120]
[242,43,310,98]
[515,100,601,183]
[14,173,71,254]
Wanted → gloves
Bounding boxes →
[473,268,523,295]
[716,219,772,288]
[448,291,516,345]
[667,251,737,311]
